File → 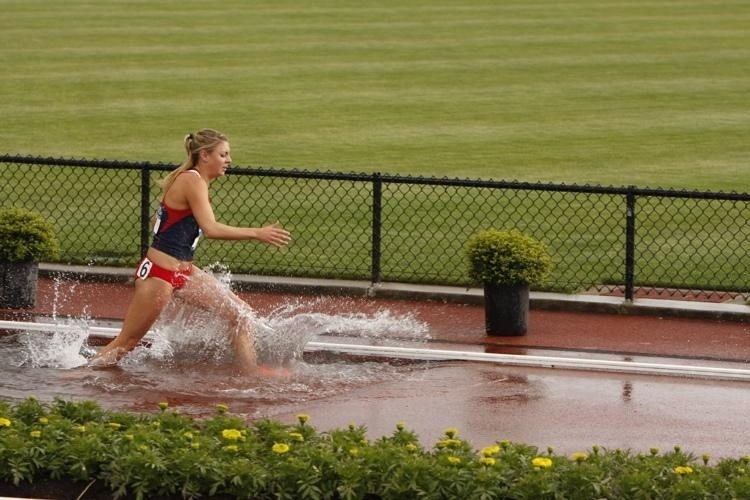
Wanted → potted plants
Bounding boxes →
[465,231,552,336]
[1,209,62,309]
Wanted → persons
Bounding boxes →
[99,128,291,369]
